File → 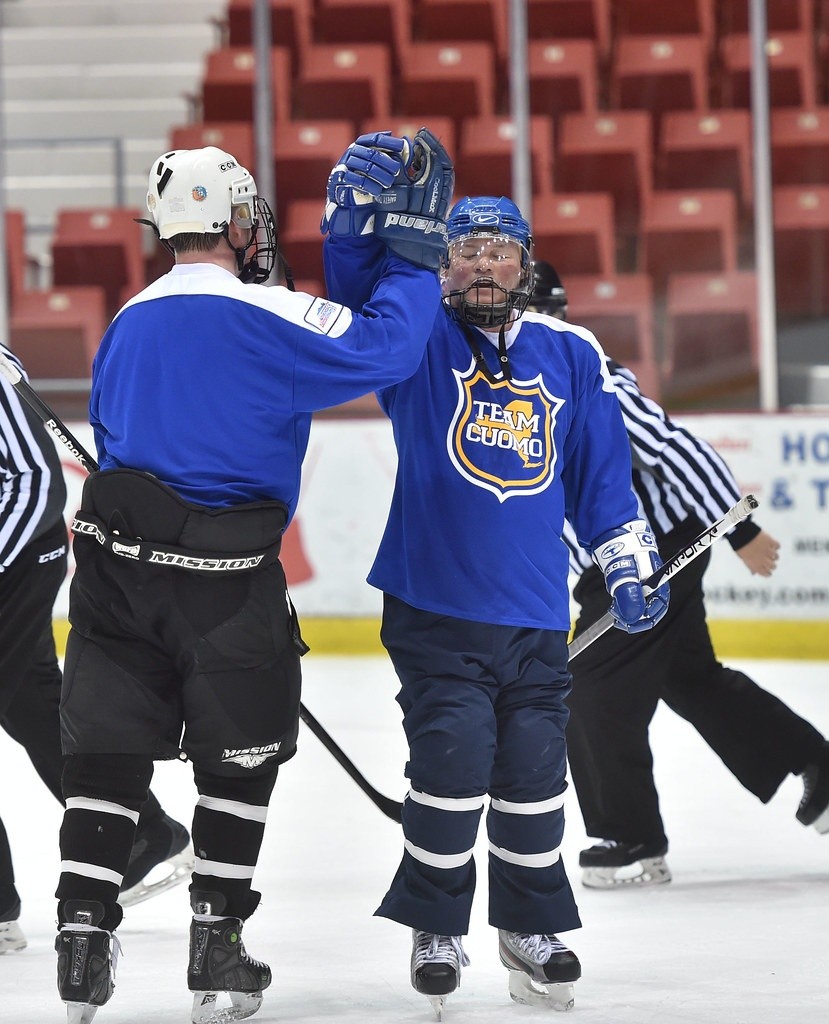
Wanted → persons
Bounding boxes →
[1,343,191,955]
[49,129,458,1024]
[514,259,829,889]
[318,129,668,1021]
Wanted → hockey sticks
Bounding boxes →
[568,493,759,664]
[3,358,402,821]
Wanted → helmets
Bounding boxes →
[147,144,259,240]
[444,194,533,267]
[514,263,568,309]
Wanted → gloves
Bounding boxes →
[591,517,670,634]
[320,132,412,238]
[373,127,455,270]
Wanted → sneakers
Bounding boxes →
[54,898,123,1024]
[0,886,26,954]
[797,743,829,836]
[187,889,272,1022]
[117,814,196,908]
[410,927,470,1022]
[579,827,670,890]
[498,928,580,1012]
